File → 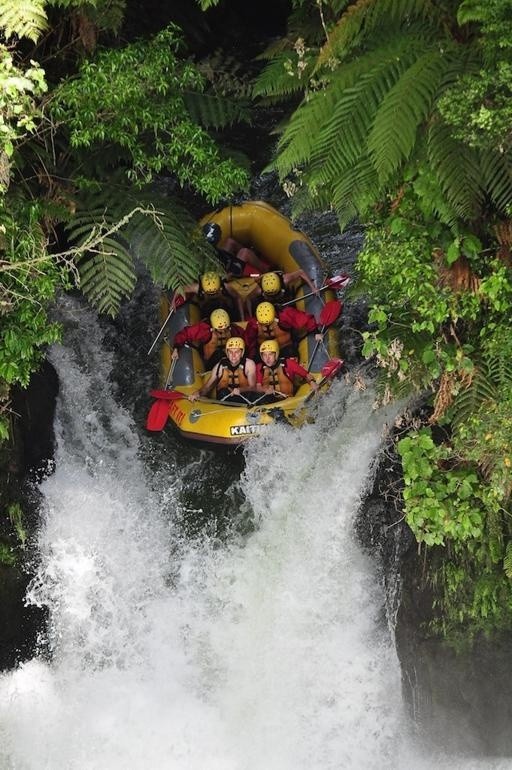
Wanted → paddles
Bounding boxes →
[145,356,177,432]
[147,292,195,357]
[303,357,345,404]
[306,300,342,373]
[148,389,248,407]
[281,272,352,307]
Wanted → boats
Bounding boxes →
[159,200,341,445]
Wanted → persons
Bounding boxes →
[245,301,323,358]
[171,308,246,365]
[255,340,317,402]
[168,270,247,325]
[240,269,319,316]
[187,336,255,402]
[202,222,271,281]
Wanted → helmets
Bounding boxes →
[201,271,220,294]
[260,340,280,361]
[225,337,245,358]
[255,302,274,325]
[262,272,280,296]
[210,309,230,330]
